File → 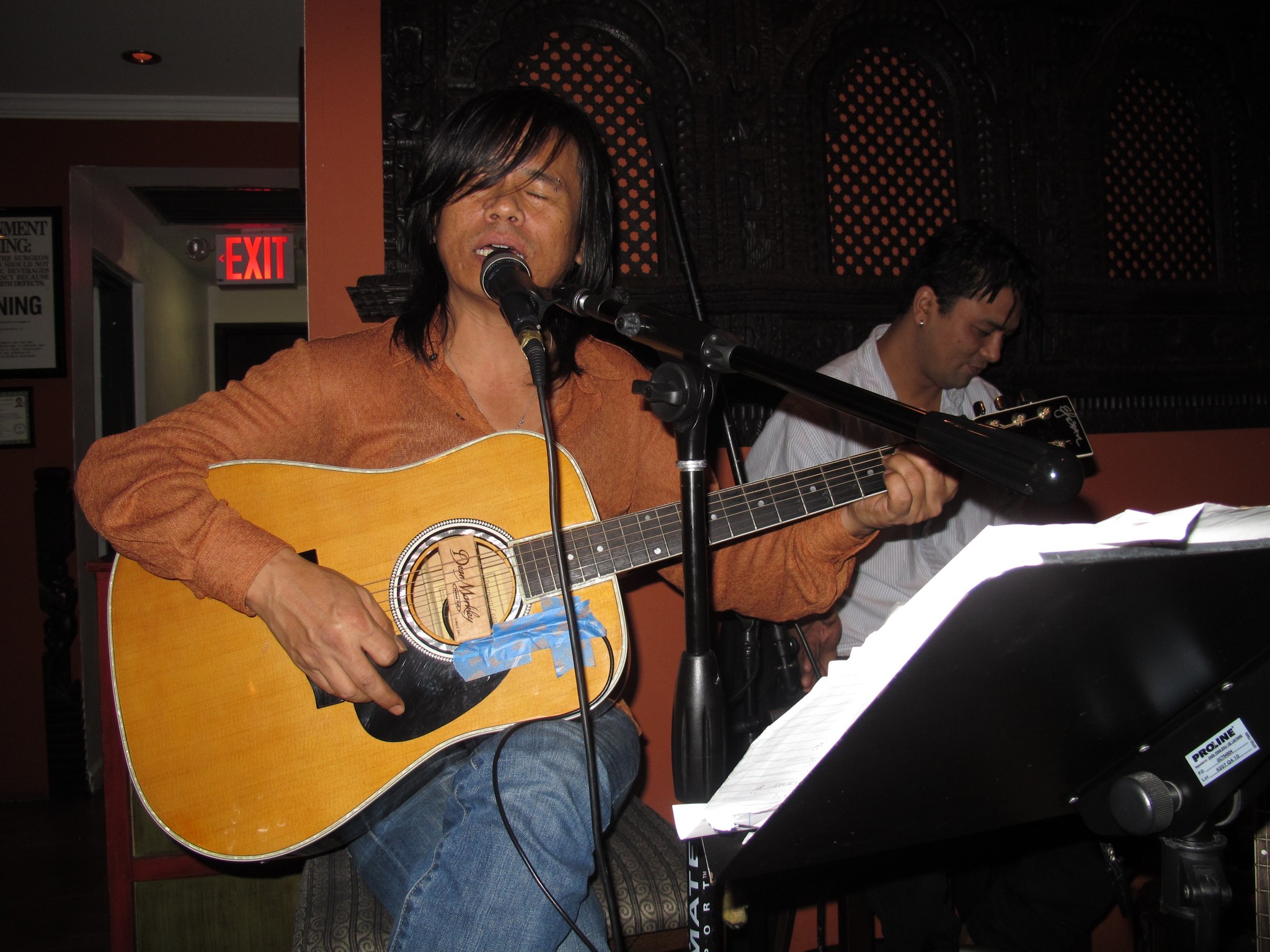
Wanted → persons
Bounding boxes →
[739,226,1070,698]
[77,80,957,952]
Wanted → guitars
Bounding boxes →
[104,395,1098,865]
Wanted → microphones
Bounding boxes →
[479,247,549,386]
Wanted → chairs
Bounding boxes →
[290,789,693,952]
